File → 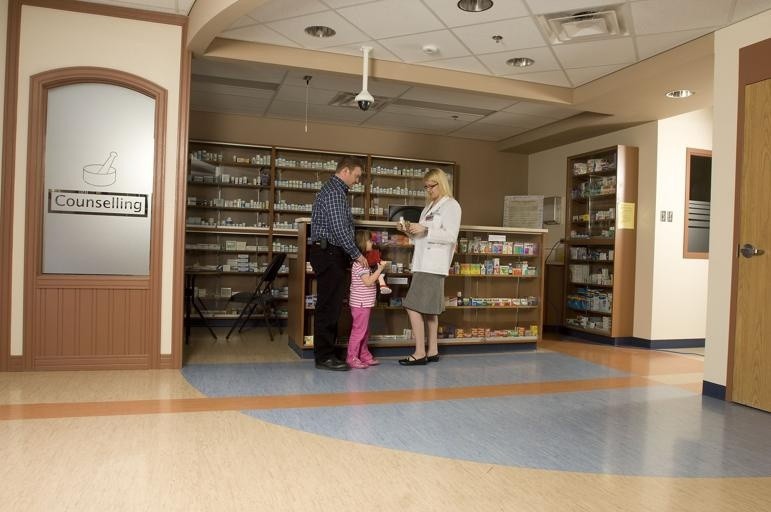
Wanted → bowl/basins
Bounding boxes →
[82,164,117,187]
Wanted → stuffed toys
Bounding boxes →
[364,249,393,295]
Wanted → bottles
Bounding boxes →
[514,261,529,276]
[456,291,463,306]
[185,148,450,253]
[253,262,289,318]
[390,262,403,274]
[481,264,486,275]
[453,260,461,275]
[437,327,444,339]
[508,262,513,275]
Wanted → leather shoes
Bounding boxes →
[315,355,350,372]
[427,354,441,362]
[397,354,428,365]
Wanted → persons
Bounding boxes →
[345,229,381,369]
[310,155,370,372]
[398,167,462,366]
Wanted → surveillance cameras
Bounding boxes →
[355,91,375,111]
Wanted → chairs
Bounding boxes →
[226,252,286,342]
[185,270,217,345]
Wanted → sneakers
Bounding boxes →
[346,359,369,370]
[361,357,382,366]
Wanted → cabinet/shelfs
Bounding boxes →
[288,217,549,360]
[187,139,272,322]
[562,144,639,338]
[272,144,369,323]
[369,155,458,219]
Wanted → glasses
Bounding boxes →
[423,182,438,189]
